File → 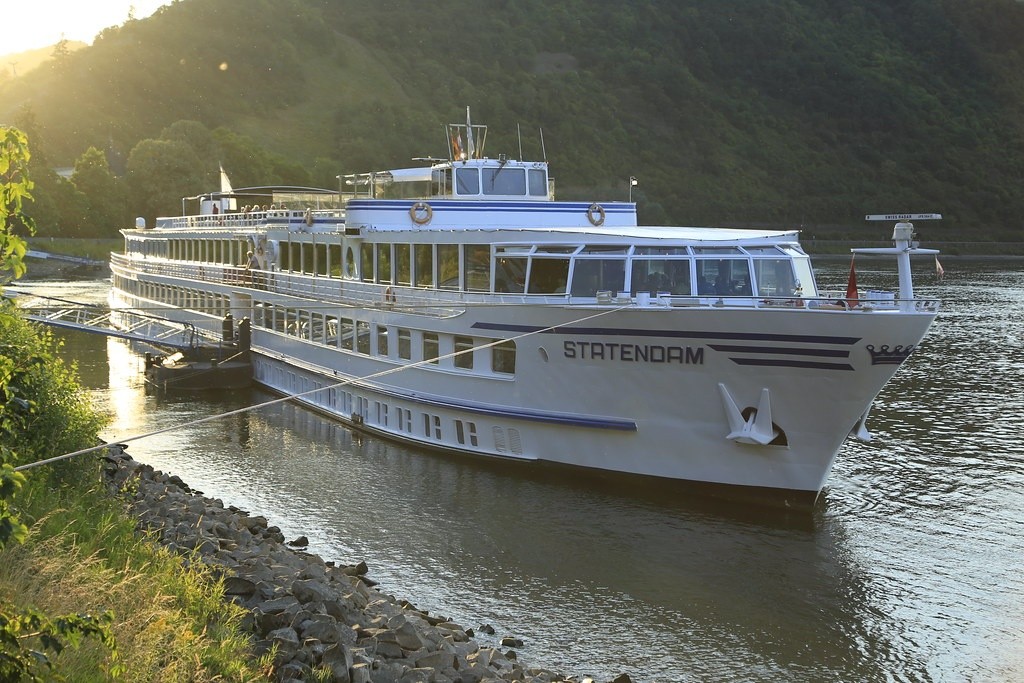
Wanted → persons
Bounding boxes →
[241,204,286,212]
[212,203,218,226]
[245,251,261,289]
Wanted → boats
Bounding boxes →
[109,101,942,518]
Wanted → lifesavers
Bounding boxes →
[386,287,395,307]
[305,208,311,226]
[410,202,432,223]
[587,204,605,225]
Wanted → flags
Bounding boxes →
[846,257,858,308]
